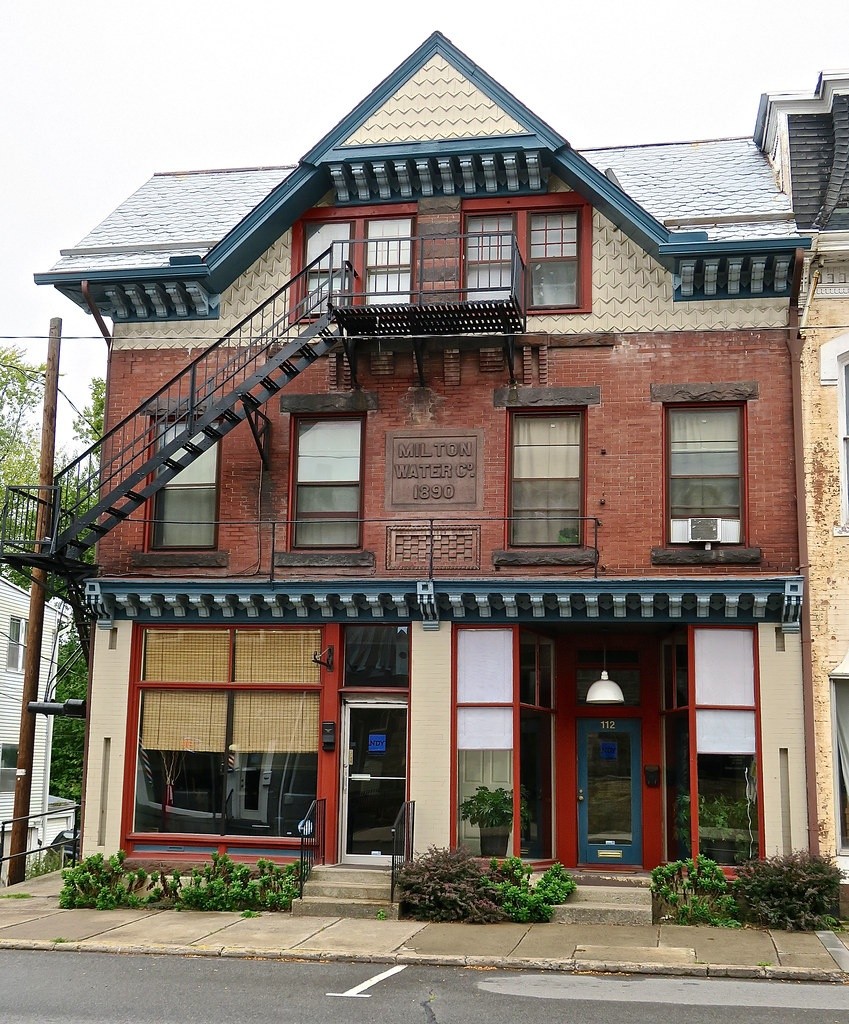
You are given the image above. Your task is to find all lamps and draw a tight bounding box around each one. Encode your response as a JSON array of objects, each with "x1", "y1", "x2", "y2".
[{"x1": 586, "y1": 625, "x2": 624, "y2": 703}]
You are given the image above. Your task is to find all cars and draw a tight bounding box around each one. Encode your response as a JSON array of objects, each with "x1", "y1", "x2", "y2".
[{"x1": 46, "y1": 830, "x2": 81, "y2": 861}]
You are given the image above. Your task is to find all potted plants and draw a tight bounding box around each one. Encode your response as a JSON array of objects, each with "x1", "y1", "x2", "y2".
[
  {"x1": 672, "y1": 794, "x2": 755, "y2": 863},
  {"x1": 459, "y1": 783, "x2": 531, "y2": 857}
]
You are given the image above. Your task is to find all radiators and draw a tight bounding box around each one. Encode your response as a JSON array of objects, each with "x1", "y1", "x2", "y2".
[{"x1": 671, "y1": 518, "x2": 740, "y2": 543}]
